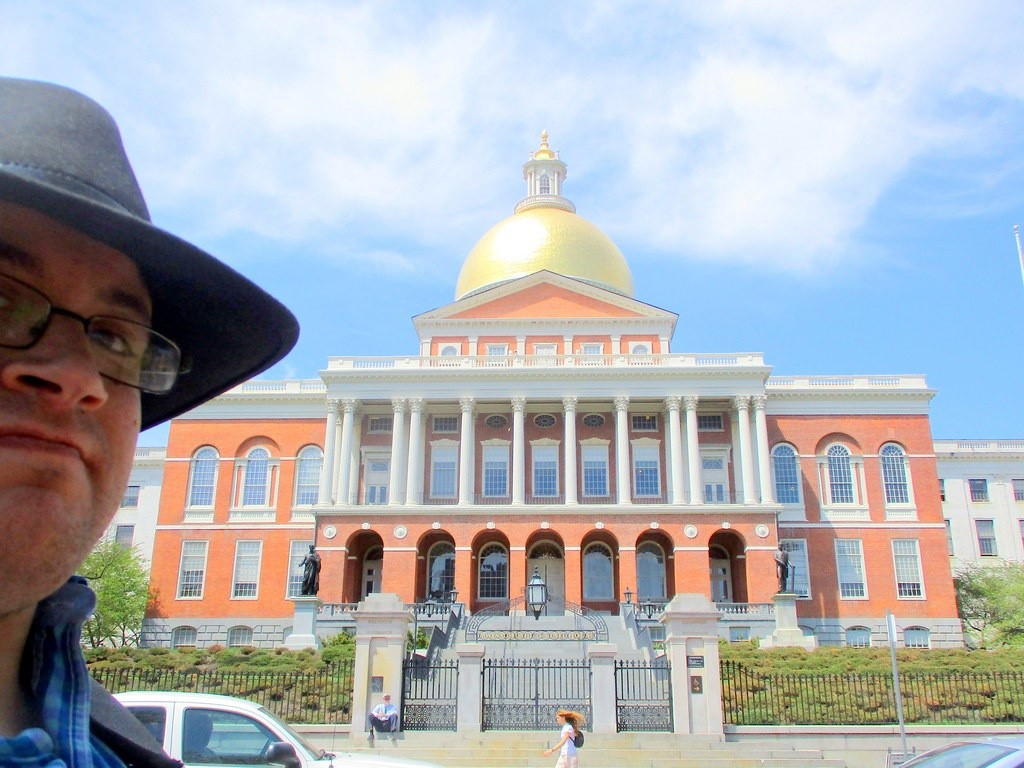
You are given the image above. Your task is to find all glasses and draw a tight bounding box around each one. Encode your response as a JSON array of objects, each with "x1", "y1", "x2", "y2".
[{"x1": 0, "y1": 272, "x2": 192, "y2": 396}]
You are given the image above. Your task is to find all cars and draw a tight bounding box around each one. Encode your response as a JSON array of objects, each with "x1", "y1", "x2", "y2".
[
  {"x1": 891, "y1": 742, "x2": 1024, "y2": 768},
  {"x1": 109, "y1": 690, "x2": 438, "y2": 768}
]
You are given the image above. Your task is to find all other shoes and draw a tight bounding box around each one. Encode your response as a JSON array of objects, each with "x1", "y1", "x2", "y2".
[
  {"x1": 367, "y1": 734, "x2": 374, "y2": 741},
  {"x1": 391, "y1": 737, "x2": 398, "y2": 741}
]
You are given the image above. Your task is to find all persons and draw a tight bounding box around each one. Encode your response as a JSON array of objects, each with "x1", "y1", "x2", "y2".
[
  {"x1": 541, "y1": 707, "x2": 587, "y2": 768},
  {"x1": 773, "y1": 541, "x2": 796, "y2": 594},
  {"x1": 1, "y1": 73, "x2": 298, "y2": 768},
  {"x1": 298, "y1": 544, "x2": 321, "y2": 596},
  {"x1": 366, "y1": 692, "x2": 399, "y2": 742}
]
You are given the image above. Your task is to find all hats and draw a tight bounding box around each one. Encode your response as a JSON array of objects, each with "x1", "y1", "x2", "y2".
[{"x1": 1, "y1": 75, "x2": 301, "y2": 437}]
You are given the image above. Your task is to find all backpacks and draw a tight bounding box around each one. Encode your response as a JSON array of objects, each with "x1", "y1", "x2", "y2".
[{"x1": 566, "y1": 724, "x2": 584, "y2": 748}]
